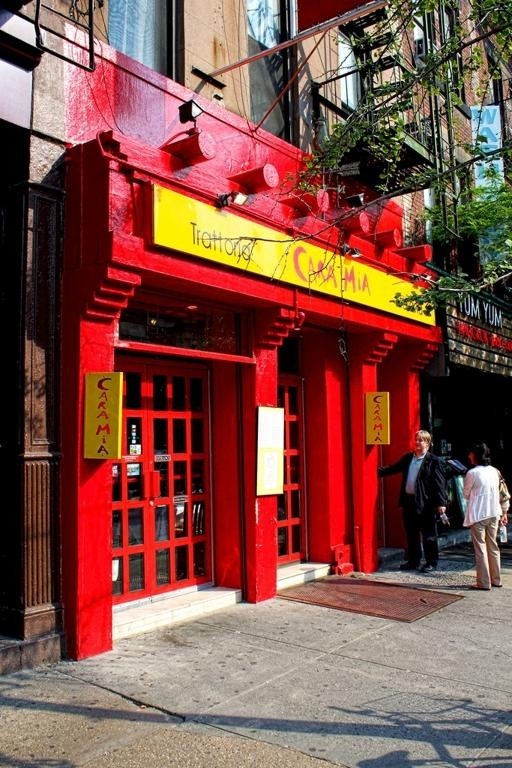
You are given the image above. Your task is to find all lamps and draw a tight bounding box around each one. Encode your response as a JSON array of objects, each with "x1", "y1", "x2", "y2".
[
  {"x1": 216, "y1": 191, "x2": 249, "y2": 208},
  {"x1": 343, "y1": 192, "x2": 364, "y2": 207},
  {"x1": 178, "y1": 99, "x2": 204, "y2": 127},
  {"x1": 340, "y1": 241, "x2": 363, "y2": 257}
]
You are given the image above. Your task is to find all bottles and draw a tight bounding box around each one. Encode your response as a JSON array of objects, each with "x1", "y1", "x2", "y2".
[
  {"x1": 499, "y1": 521, "x2": 509, "y2": 544},
  {"x1": 440, "y1": 510, "x2": 450, "y2": 528}
]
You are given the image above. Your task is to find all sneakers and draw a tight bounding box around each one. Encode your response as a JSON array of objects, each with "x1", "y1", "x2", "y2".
[{"x1": 469, "y1": 583, "x2": 503, "y2": 592}]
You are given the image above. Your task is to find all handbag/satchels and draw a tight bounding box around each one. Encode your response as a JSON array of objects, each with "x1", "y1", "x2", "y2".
[{"x1": 497, "y1": 469, "x2": 512, "y2": 504}]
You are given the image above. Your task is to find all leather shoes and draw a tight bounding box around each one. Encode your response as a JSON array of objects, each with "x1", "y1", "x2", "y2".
[{"x1": 398, "y1": 562, "x2": 436, "y2": 575}]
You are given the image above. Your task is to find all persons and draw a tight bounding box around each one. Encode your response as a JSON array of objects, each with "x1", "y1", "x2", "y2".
[
  {"x1": 378, "y1": 430, "x2": 450, "y2": 571},
  {"x1": 462, "y1": 442, "x2": 511, "y2": 590}
]
